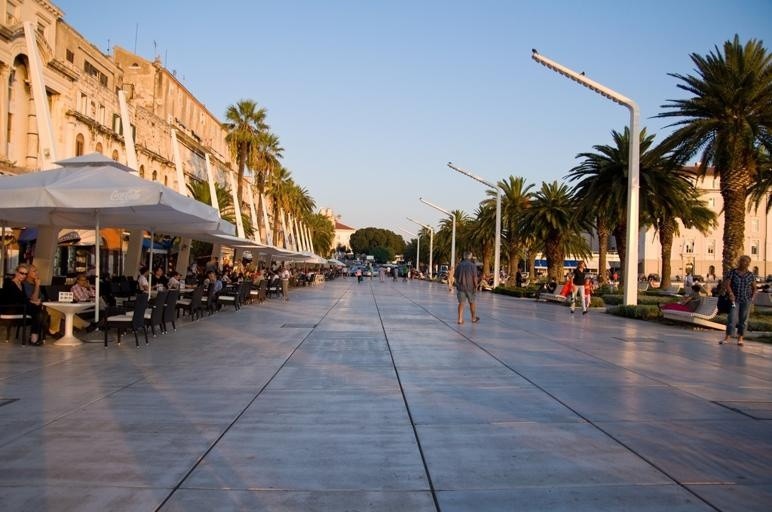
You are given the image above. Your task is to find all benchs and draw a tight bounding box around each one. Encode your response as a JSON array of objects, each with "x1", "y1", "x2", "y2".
[
  {"x1": 539, "y1": 285, "x2": 567, "y2": 302},
  {"x1": 662, "y1": 296, "x2": 727, "y2": 330},
  {"x1": 754, "y1": 293, "x2": 772, "y2": 307}
]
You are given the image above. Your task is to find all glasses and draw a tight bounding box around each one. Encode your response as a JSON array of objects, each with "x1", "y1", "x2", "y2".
[{"x1": 17, "y1": 271, "x2": 28, "y2": 275}]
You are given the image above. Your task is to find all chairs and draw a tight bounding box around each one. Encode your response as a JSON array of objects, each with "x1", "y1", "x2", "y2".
[{"x1": 0, "y1": 270, "x2": 313, "y2": 352}]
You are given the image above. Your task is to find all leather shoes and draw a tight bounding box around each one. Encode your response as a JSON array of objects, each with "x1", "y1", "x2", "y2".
[{"x1": 30, "y1": 320, "x2": 105, "y2": 346}]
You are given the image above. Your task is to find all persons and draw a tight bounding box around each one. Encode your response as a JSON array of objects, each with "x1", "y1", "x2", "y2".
[
  {"x1": 445, "y1": 264, "x2": 455, "y2": 292},
  {"x1": 516, "y1": 253, "x2": 772, "y2": 347},
  {"x1": 338, "y1": 265, "x2": 374, "y2": 282},
  {"x1": 455, "y1": 250, "x2": 480, "y2": 325},
  {"x1": 246, "y1": 264, "x2": 333, "y2": 300},
  {"x1": 71, "y1": 265, "x2": 106, "y2": 331},
  {"x1": 189, "y1": 261, "x2": 244, "y2": 309},
  {"x1": 137, "y1": 266, "x2": 192, "y2": 292},
  {"x1": 379, "y1": 266, "x2": 416, "y2": 282},
  {"x1": 3, "y1": 265, "x2": 44, "y2": 346}
]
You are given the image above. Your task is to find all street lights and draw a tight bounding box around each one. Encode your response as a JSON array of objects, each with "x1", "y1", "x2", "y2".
[
  {"x1": 396, "y1": 225, "x2": 420, "y2": 272},
  {"x1": 419, "y1": 196, "x2": 456, "y2": 275},
  {"x1": 529, "y1": 47, "x2": 644, "y2": 308},
  {"x1": 405, "y1": 216, "x2": 434, "y2": 279},
  {"x1": 445, "y1": 160, "x2": 502, "y2": 289}
]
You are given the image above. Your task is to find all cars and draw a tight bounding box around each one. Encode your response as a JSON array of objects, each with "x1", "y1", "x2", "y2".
[
  {"x1": 343, "y1": 260, "x2": 449, "y2": 279},
  {"x1": 585, "y1": 271, "x2": 597, "y2": 278}
]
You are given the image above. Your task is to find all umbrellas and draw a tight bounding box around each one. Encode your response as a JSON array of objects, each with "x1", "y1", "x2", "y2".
[{"x1": 0, "y1": 151, "x2": 222, "y2": 323}]
